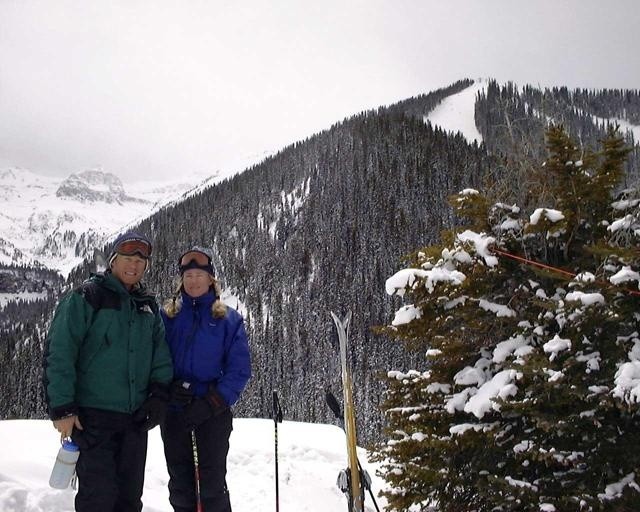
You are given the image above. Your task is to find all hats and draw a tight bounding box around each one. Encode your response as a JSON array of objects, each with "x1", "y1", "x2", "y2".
[
  {"x1": 110, "y1": 233, "x2": 151, "y2": 272},
  {"x1": 180, "y1": 259, "x2": 215, "y2": 277}
]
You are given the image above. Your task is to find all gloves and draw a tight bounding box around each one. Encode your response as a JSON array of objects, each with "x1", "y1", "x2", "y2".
[
  {"x1": 180, "y1": 380, "x2": 230, "y2": 428},
  {"x1": 170, "y1": 379, "x2": 194, "y2": 406},
  {"x1": 130, "y1": 384, "x2": 174, "y2": 433}
]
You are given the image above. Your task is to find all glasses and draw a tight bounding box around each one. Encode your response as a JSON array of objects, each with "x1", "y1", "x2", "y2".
[
  {"x1": 116, "y1": 238, "x2": 151, "y2": 258},
  {"x1": 180, "y1": 251, "x2": 210, "y2": 267}
]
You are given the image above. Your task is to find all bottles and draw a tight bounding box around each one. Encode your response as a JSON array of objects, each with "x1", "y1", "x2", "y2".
[{"x1": 49, "y1": 442, "x2": 81, "y2": 490}]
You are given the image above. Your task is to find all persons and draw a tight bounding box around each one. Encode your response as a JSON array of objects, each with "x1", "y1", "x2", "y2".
[
  {"x1": 150, "y1": 245, "x2": 253, "y2": 512},
  {"x1": 41, "y1": 230, "x2": 176, "y2": 511}
]
